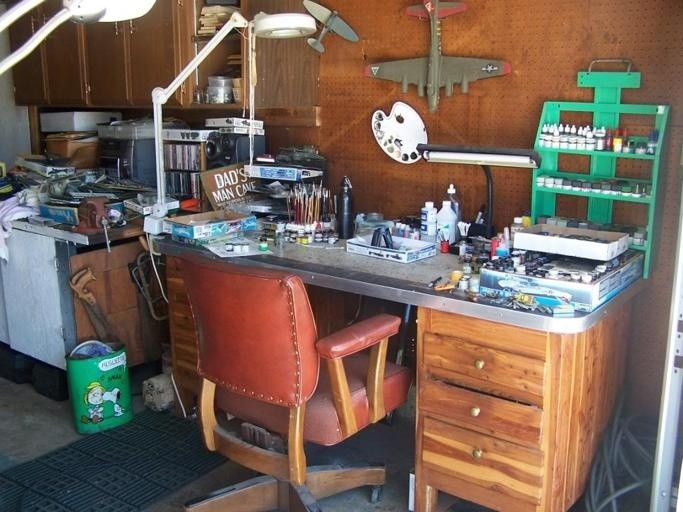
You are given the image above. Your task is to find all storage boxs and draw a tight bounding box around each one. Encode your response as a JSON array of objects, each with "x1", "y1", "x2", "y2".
[{"x1": 45, "y1": 132, "x2": 98, "y2": 169}]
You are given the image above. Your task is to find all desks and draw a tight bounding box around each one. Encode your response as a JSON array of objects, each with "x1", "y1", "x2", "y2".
[
  {"x1": 153, "y1": 232, "x2": 653, "y2": 512},
  {"x1": 1, "y1": 223, "x2": 167, "y2": 375}
]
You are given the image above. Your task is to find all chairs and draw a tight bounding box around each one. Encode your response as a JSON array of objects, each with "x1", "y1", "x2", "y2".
[{"x1": 181, "y1": 254, "x2": 411, "y2": 511}]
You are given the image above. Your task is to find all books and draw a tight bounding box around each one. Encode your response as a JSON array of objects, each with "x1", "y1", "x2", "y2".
[{"x1": 162, "y1": 143, "x2": 201, "y2": 201}]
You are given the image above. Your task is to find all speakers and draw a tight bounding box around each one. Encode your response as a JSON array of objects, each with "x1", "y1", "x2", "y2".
[{"x1": 204, "y1": 131, "x2": 266, "y2": 171}]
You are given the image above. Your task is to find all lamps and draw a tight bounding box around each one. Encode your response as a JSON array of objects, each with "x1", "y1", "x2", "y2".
[
  {"x1": 416, "y1": 143, "x2": 542, "y2": 239},
  {"x1": 142, "y1": 10, "x2": 319, "y2": 235}
]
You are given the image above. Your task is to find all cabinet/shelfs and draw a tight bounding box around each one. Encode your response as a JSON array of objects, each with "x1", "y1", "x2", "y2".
[
  {"x1": 165, "y1": 255, "x2": 365, "y2": 417},
  {"x1": 13, "y1": 0, "x2": 185, "y2": 108},
  {"x1": 414, "y1": 306, "x2": 633, "y2": 512},
  {"x1": 190, "y1": 0, "x2": 250, "y2": 110}
]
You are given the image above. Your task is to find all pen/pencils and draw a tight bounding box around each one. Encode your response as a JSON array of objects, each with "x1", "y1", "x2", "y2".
[
  {"x1": 428, "y1": 277, "x2": 442, "y2": 287},
  {"x1": 288, "y1": 180, "x2": 332, "y2": 223}
]
